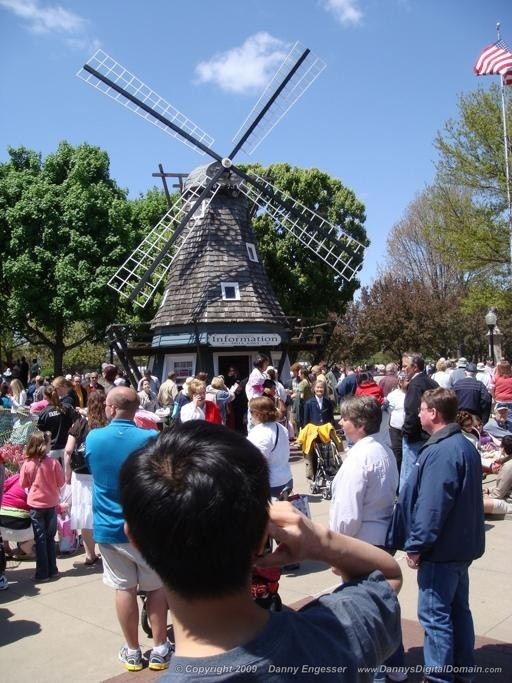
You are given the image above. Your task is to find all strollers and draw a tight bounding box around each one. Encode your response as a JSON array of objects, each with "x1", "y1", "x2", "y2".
[{"x1": 309, "y1": 422, "x2": 343, "y2": 501}]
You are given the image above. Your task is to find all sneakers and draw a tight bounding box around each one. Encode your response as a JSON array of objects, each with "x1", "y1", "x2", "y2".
[
  {"x1": 148, "y1": 649, "x2": 173, "y2": 671},
  {"x1": 116, "y1": 644, "x2": 142, "y2": 671},
  {"x1": 0, "y1": 577, "x2": 8, "y2": 589},
  {"x1": 31, "y1": 576, "x2": 49, "y2": 581}
]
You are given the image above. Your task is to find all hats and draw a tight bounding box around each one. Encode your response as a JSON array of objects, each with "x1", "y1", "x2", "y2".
[
  {"x1": 457, "y1": 362, "x2": 483, "y2": 374},
  {"x1": 477, "y1": 362, "x2": 486, "y2": 371},
  {"x1": 457, "y1": 357, "x2": 466, "y2": 367},
  {"x1": 495, "y1": 403, "x2": 508, "y2": 411},
  {"x1": 102, "y1": 362, "x2": 119, "y2": 377}
]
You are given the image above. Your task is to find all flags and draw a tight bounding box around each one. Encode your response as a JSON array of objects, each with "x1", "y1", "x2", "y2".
[{"x1": 473, "y1": 39, "x2": 512, "y2": 85}]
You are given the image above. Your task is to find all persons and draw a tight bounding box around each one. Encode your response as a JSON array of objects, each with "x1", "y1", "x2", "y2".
[
  {"x1": 0, "y1": 355, "x2": 105, "y2": 592},
  {"x1": 133, "y1": 394, "x2": 166, "y2": 432},
  {"x1": 97, "y1": 362, "x2": 130, "y2": 396},
  {"x1": 329, "y1": 361, "x2": 402, "y2": 395},
  {"x1": 402, "y1": 351, "x2": 512, "y2": 683},
  {"x1": 85, "y1": 387, "x2": 176, "y2": 671},
  {"x1": 290, "y1": 360, "x2": 334, "y2": 429},
  {"x1": 137, "y1": 379, "x2": 160, "y2": 414},
  {"x1": 329, "y1": 394, "x2": 408, "y2": 683},
  {"x1": 157, "y1": 371, "x2": 179, "y2": 431},
  {"x1": 175, "y1": 368, "x2": 247, "y2": 424},
  {"x1": 137, "y1": 369, "x2": 160, "y2": 396},
  {"x1": 118, "y1": 420, "x2": 402, "y2": 682},
  {"x1": 247, "y1": 397, "x2": 301, "y2": 572},
  {"x1": 245, "y1": 352, "x2": 290, "y2": 403}
]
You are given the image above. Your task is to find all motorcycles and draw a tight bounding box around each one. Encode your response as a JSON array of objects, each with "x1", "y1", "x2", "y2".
[
  {"x1": 142, "y1": 537, "x2": 283, "y2": 635},
  {"x1": 271, "y1": 486, "x2": 311, "y2": 570}
]
